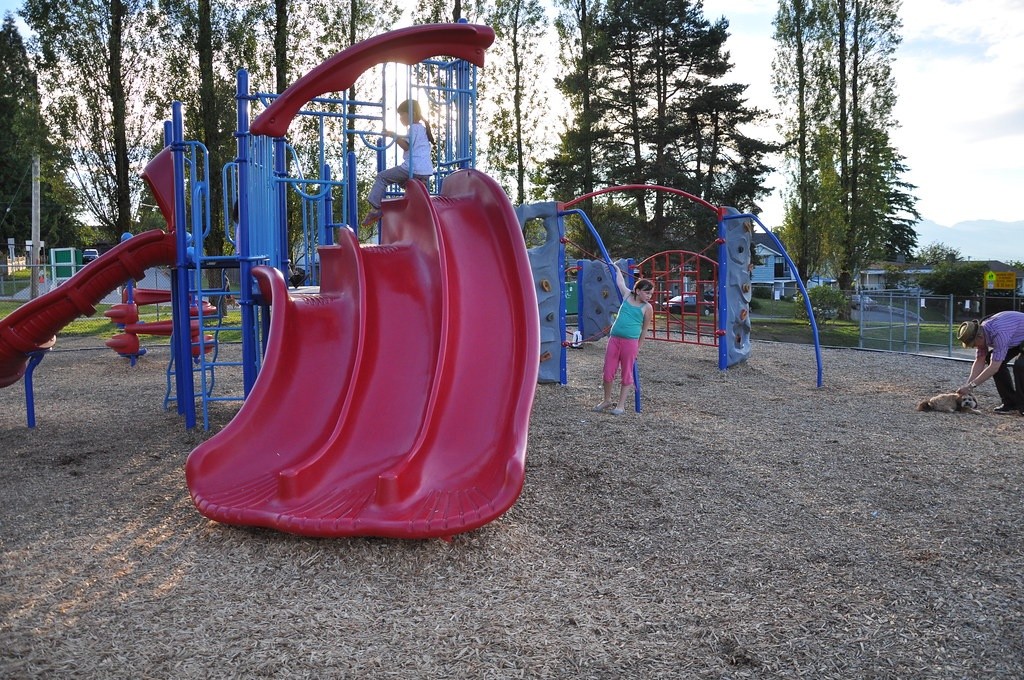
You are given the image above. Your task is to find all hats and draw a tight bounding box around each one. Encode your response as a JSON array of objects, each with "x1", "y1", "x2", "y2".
[{"x1": 956, "y1": 318, "x2": 979, "y2": 349}]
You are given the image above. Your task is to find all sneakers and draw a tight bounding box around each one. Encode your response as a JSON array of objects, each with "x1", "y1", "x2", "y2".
[
  {"x1": 361, "y1": 208, "x2": 384, "y2": 227},
  {"x1": 1020, "y1": 409, "x2": 1024, "y2": 415},
  {"x1": 993, "y1": 404, "x2": 1019, "y2": 414}
]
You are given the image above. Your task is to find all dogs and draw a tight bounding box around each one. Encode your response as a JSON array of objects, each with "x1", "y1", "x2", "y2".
[
  {"x1": 916, "y1": 392, "x2": 982, "y2": 415},
  {"x1": 226, "y1": 299, "x2": 239, "y2": 309}
]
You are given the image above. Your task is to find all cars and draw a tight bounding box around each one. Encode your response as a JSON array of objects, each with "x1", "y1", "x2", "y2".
[
  {"x1": 663, "y1": 295, "x2": 714, "y2": 316},
  {"x1": 82, "y1": 249, "x2": 99, "y2": 264},
  {"x1": 846, "y1": 295, "x2": 878, "y2": 311}
]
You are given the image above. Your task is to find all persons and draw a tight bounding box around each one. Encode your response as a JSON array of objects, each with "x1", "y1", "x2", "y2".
[
  {"x1": 362, "y1": 98, "x2": 436, "y2": 226},
  {"x1": 955, "y1": 311, "x2": 1024, "y2": 417},
  {"x1": 591, "y1": 260, "x2": 654, "y2": 415}
]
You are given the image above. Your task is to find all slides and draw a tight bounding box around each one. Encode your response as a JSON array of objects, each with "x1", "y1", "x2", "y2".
[
  {"x1": 186, "y1": 167, "x2": 540, "y2": 533},
  {"x1": 0, "y1": 223, "x2": 173, "y2": 388}
]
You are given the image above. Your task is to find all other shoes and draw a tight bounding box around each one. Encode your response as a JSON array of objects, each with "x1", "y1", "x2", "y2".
[
  {"x1": 591, "y1": 400, "x2": 611, "y2": 411},
  {"x1": 611, "y1": 407, "x2": 626, "y2": 414}
]
